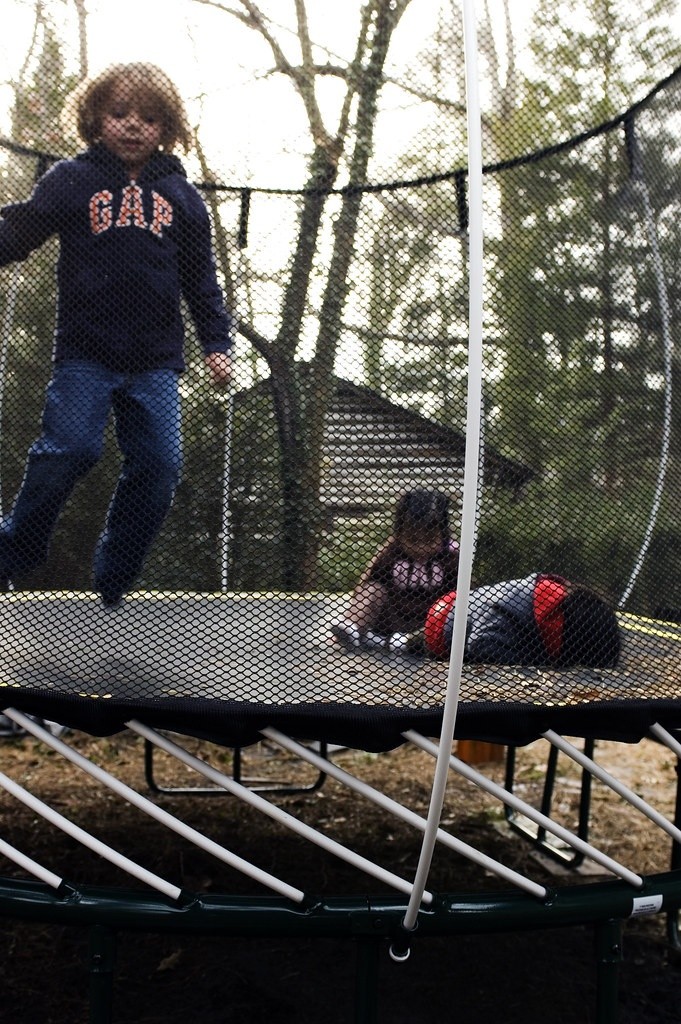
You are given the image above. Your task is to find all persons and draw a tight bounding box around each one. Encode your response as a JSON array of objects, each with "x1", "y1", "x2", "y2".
[
  {"x1": 332, "y1": 490, "x2": 621, "y2": 669},
  {"x1": 0, "y1": 61, "x2": 235, "y2": 609}
]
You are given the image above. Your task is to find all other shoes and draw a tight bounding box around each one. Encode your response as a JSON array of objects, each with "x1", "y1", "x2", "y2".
[
  {"x1": 331, "y1": 621, "x2": 361, "y2": 651},
  {"x1": 384, "y1": 633, "x2": 407, "y2": 653}
]
